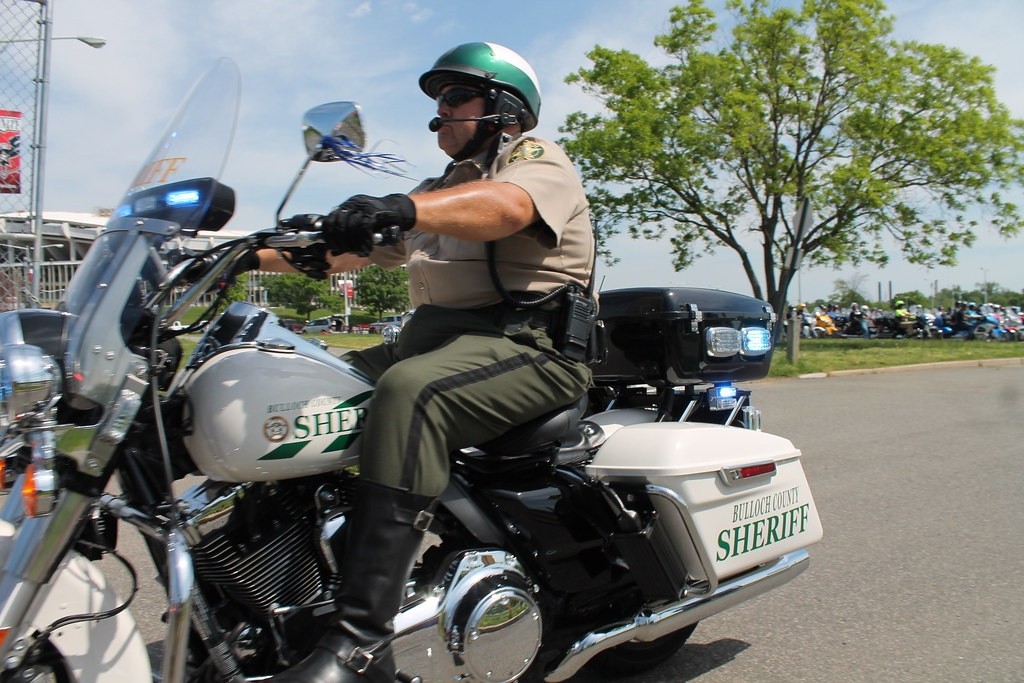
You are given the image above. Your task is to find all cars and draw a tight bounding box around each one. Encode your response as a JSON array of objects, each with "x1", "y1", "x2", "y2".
[
  {"x1": 302, "y1": 313, "x2": 352, "y2": 334},
  {"x1": 369, "y1": 314, "x2": 402, "y2": 334},
  {"x1": 279, "y1": 318, "x2": 304, "y2": 334}
]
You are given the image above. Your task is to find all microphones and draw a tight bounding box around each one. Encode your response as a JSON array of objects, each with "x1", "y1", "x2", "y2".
[{"x1": 429, "y1": 113, "x2": 509, "y2": 133}]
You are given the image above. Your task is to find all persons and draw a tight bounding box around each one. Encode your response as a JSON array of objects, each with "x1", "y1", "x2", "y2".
[
  {"x1": 169, "y1": 41, "x2": 601, "y2": 683},
  {"x1": 783, "y1": 300, "x2": 1024, "y2": 345}
]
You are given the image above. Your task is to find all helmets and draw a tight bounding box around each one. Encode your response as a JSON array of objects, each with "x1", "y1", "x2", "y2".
[
  {"x1": 795, "y1": 298, "x2": 1023, "y2": 319},
  {"x1": 418, "y1": 42, "x2": 542, "y2": 133}
]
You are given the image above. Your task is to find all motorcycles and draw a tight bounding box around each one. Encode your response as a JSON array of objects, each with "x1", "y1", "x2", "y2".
[
  {"x1": 783, "y1": 303, "x2": 1024, "y2": 344},
  {"x1": 0, "y1": 51, "x2": 825, "y2": 683}
]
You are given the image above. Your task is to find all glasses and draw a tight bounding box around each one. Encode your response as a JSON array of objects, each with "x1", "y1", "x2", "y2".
[{"x1": 436, "y1": 87, "x2": 490, "y2": 107}]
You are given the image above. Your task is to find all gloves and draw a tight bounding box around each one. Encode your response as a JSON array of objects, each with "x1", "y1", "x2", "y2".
[
  {"x1": 322, "y1": 194, "x2": 417, "y2": 256},
  {"x1": 206, "y1": 248, "x2": 260, "y2": 284}
]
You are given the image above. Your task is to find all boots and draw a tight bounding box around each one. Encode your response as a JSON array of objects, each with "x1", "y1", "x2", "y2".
[{"x1": 267, "y1": 476, "x2": 439, "y2": 683}]
